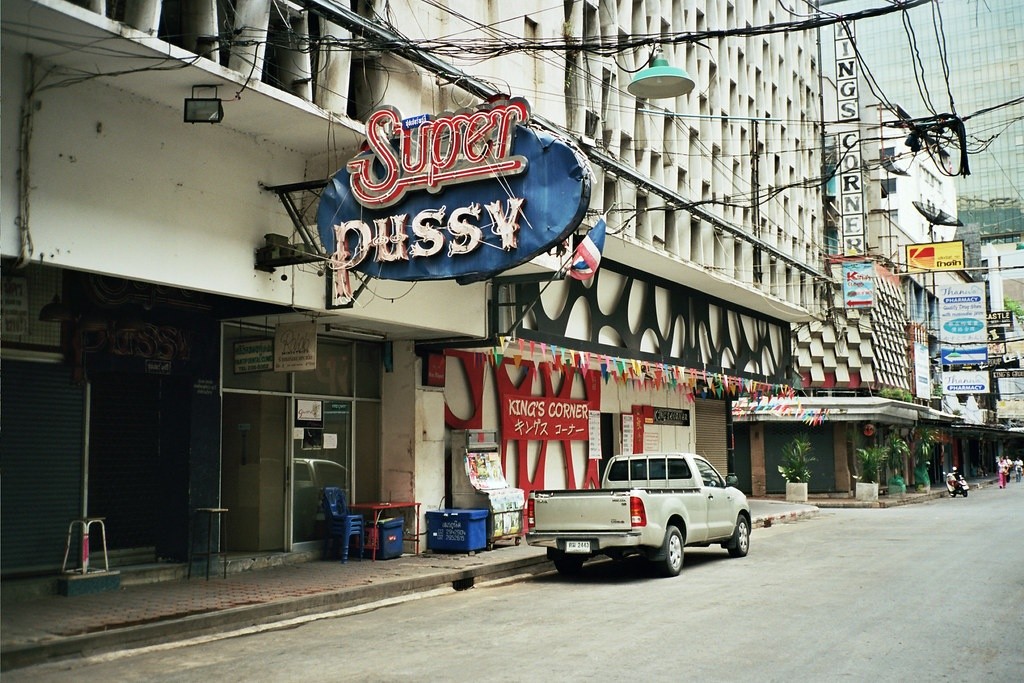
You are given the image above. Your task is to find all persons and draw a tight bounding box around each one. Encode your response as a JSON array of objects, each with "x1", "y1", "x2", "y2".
[
  {"x1": 998, "y1": 456, "x2": 1009, "y2": 488},
  {"x1": 1014, "y1": 457, "x2": 1023, "y2": 483},
  {"x1": 1003, "y1": 456, "x2": 1013, "y2": 483}
]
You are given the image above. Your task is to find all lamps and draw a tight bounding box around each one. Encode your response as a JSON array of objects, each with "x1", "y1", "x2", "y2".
[
  {"x1": 627, "y1": 43, "x2": 695, "y2": 98},
  {"x1": 183, "y1": 84, "x2": 225, "y2": 125}
]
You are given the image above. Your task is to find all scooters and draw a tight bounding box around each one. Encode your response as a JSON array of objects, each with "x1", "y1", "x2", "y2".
[{"x1": 942, "y1": 467, "x2": 969, "y2": 497}]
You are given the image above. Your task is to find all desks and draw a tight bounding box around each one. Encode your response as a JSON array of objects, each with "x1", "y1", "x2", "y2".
[{"x1": 347, "y1": 503, "x2": 420, "y2": 562}]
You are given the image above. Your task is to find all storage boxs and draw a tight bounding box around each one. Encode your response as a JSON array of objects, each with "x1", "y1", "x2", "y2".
[
  {"x1": 427, "y1": 508, "x2": 488, "y2": 551},
  {"x1": 353, "y1": 518, "x2": 405, "y2": 560}
]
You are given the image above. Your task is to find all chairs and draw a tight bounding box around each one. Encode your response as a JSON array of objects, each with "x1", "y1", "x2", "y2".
[{"x1": 321, "y1": 485, "x2": 365, "y2": 564}]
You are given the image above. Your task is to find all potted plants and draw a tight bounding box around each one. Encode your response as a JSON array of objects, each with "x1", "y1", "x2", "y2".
[
  {"x1": 912, "y1": 426, "x2": 940, "y2": 493},
  {"x1": 884, "y1": 432, "x2": 913, "y2": 498},
  {"x1": 776, "y1": 431, "x2": 817, "y2": 501},
  {"x1": 843, "y1": 428, "x2": 891, "y2": 501}
]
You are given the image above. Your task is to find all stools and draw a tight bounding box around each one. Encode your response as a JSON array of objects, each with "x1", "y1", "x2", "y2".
[
  {"x1": 187, "y1": 507, "x2": 230, "y2": 580},
  {"x1": 61, "y1": 516, "x2": 109, "y2": 575}
]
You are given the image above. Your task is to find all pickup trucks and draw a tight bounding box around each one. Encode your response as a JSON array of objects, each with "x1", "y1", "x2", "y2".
[
  {"x1": 525, "y1": 451, "x2": 754, "y2": 578},
  {"x1": 188, "y1": 457, "x2": 378, "y2": 533}
]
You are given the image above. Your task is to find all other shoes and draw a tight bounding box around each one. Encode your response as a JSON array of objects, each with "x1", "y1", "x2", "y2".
[{"x1": 1000, "y1": 485, "x2": 1002, "y2": 488}]
